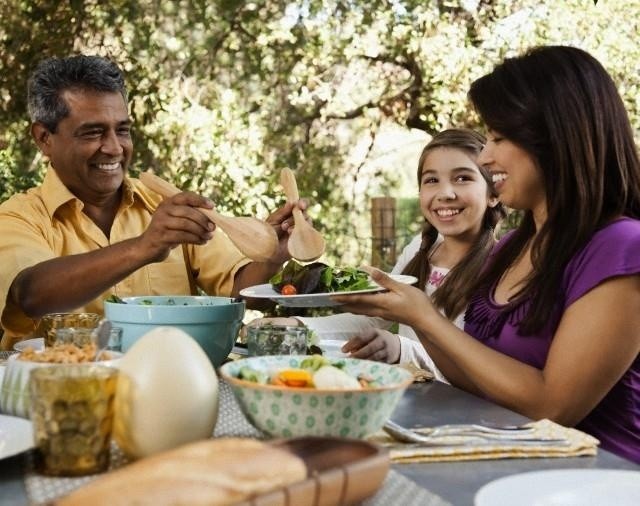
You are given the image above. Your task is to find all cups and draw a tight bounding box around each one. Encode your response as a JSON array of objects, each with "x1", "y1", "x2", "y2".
[
  {"x1": 247, "y1": 322, "x2": 309, "y2": 356},
  {"x1": 27, "y1": 363, "x2": 122, "y2": 475},
  {"x1": 40, "y1": 311, "x2": 100, "y2": 348}
]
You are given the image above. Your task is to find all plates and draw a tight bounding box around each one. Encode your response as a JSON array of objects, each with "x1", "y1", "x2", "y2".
[
  {"x1": 0, "y1": 409, "x2": 35, "y2": 463},
  {"x1": 11, "y1": 333, "x2": 45, "y2": 354},
  {"x1": 238, "y1": 272, "x2": 420, "y2": 309}
]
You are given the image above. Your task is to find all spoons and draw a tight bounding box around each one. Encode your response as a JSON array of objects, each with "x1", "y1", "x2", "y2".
[{"x1": 383, "y1": 419, "x2": 573, "y2": 448}]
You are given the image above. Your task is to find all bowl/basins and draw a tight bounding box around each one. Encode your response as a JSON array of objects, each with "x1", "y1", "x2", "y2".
[
  {"x1": 0, "y1": 350, "x2": 126, "y2": 420},
  {"x1": 103, "y1": 291, "x2": 247, "y2": 371},
  {"x1": 217, "y1": 351, "x2": 416, "y2": 440}
]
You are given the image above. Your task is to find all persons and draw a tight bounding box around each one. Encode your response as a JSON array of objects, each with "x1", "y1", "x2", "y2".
[
  {"x1": 236, "y1": 128, "x2": 507, "y2": 387},
  {"x1": 329, "y1": 46, "x2": 640, "y2": 464},
  {"x1": 0, "y1": 53, "x2": 310, "y2": 358}
]
